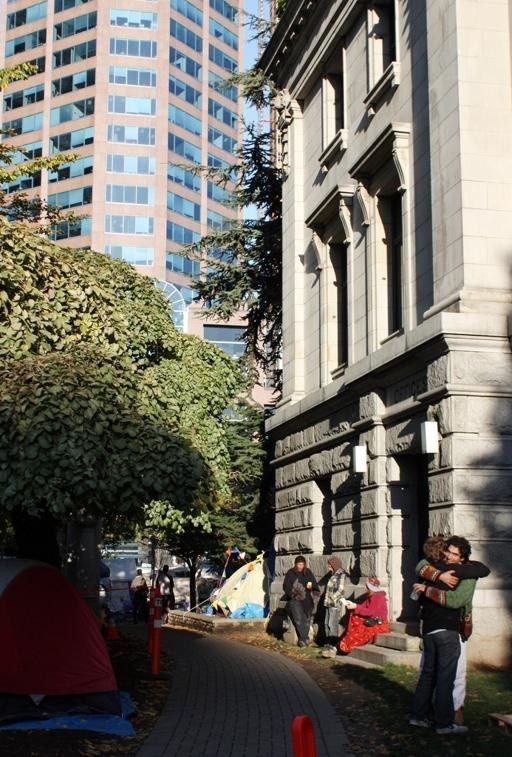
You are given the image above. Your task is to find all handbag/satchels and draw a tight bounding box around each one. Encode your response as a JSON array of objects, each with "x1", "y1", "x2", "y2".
[{"x1": 292, "y1": 573, "x2": 306, "y2": 600}]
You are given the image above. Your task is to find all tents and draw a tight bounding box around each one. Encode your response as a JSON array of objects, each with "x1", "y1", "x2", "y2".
[
  {"x1": 203, "y1": 548, "x2": 272, "y2": 618},
  {"x1": 2, "y1": 555, "x2": 135, "y2": 738}
]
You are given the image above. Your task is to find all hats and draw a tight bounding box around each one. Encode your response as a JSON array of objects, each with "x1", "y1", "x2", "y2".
[{"x1": 366, "y1": 577, "x2": 382, "y2": 592}]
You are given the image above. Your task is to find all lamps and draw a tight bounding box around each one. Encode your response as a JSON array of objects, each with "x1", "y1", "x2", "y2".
[
  {"x1": 420, "y1": 420, "x2": 442, "y2": 454},
  {"x1": 353, "y1": 445, "x2": 370, "y2": 473}
]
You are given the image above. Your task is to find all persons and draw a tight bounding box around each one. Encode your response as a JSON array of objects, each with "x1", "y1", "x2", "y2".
[
  {"x1": 196, "y1": 577, "x2": 211, "y2": 607},
  {"x1": 100, "y1": 614, "x2": 121, "y2": 639},
  {"x1": 321, "y1": 575, "x2": 390, "y2": 658},
  {"x1": 316, "y1": 556, "x2": 346, "y2": 654},
  {"x1": 406, "y1": 534, "x2": 491, "y2": 734},
  {"x1": 130, "y1": 562, "x2": 177, "y2": 626},
  {"x1": 410, "y1": 533, "x2": 477, "y2": 726},
  {"x1": 281, "y1": 554, "x2": 321, "y2": 647}
]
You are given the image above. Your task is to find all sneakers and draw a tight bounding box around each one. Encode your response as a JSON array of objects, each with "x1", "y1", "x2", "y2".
[
  {"x1": 318, "y1": 642, "x2": 338, "y2": 659},
  {"x1": 453, "y1": 707, "x2": 465, "y2": 726},
  {"x1": 436, "y1": 722, "x2": 469, "y2": 735},
  {"x1": 409, "y1": 716, "x2": 434, "y2": 728},
  {"x1": 298, "y1": 637, "x2": 310, "y2": 648}
]
